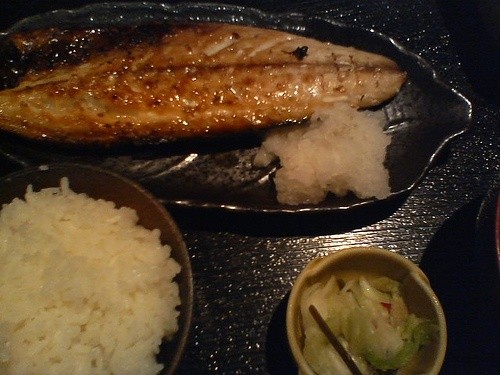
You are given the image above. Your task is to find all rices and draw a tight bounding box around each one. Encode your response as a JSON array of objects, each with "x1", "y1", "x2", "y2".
[
  {"x1": 253, "y1": 103, "x2": 393, "y2": 206},
  {"x1": 0, "y1": 177, "x2": 182, "y2": 375}
]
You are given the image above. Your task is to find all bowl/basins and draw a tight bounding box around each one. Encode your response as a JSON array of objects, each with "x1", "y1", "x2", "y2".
[
  {"x1": 1, "y1": 162, "x2": 194, "y2": 375},
  {"x1": 286, "y1": 248, "x2": 447, "y2": 375}
]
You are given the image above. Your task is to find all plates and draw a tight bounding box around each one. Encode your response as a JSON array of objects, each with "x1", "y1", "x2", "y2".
[
  {"x1": 478, "y1": 170, "x2": 500, "y2": 301},
  {"x1": 1, "y1": 1, "x2": 473, "y2": 216}
]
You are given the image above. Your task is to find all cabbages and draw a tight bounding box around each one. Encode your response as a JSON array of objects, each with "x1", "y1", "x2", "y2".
[{"x1": 299, "y1": 272, "x2": 438, "y2": 375}]
